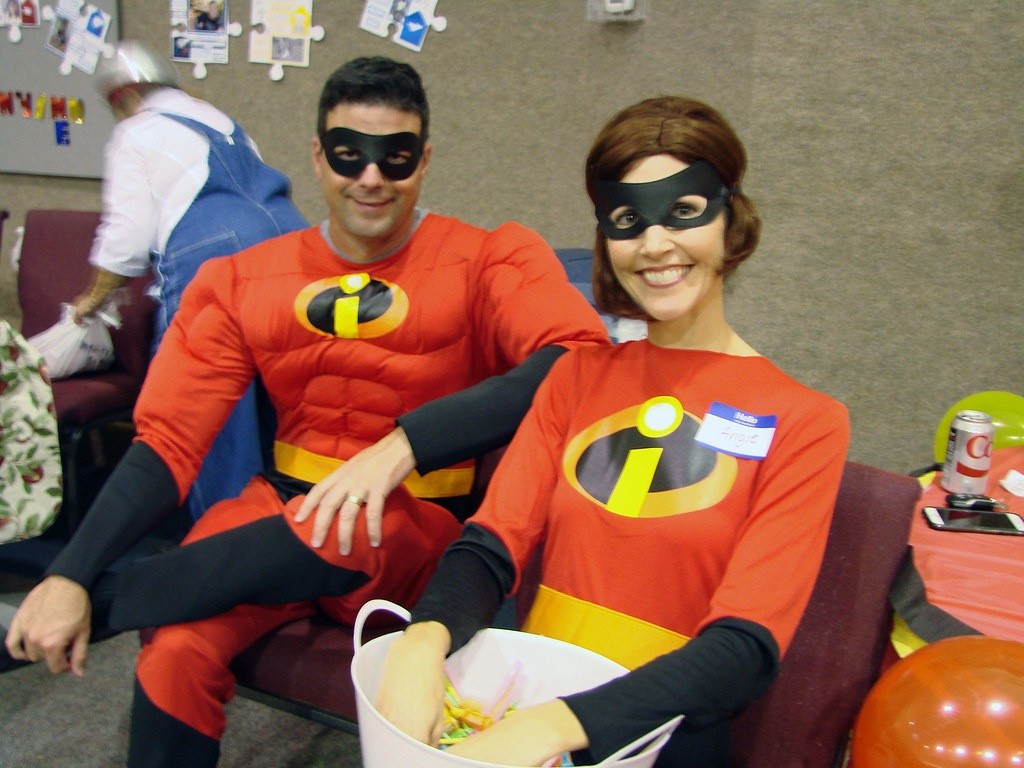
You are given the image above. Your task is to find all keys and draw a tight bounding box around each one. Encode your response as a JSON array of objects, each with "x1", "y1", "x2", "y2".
[{"x1": 946, "y1": 493, "x2": 998, "y2": 511}]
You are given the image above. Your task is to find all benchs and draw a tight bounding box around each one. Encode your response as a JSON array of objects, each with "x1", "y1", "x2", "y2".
[{"x1": 139, "y1": 445, "x2": 923, "y2": 767}]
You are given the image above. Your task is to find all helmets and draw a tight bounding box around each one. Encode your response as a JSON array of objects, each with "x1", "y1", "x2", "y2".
[{"x1": 94, "y1": 37, "x2": 180, "y2": 98}]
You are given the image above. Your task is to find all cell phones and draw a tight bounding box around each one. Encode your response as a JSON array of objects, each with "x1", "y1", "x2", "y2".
[{"x1": 923, "y1": 506, "x2": 1024, "y2": 536}]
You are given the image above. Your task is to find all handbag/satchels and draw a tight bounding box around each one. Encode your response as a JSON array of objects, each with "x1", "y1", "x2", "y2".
[{"x1": 27, "y1": 300, "x2": 121, "y2": 378}]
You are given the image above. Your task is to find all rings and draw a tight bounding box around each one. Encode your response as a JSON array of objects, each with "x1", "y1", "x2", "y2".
[{"x1": 346, "y1": 494, "x2": 363, "y2": 505}]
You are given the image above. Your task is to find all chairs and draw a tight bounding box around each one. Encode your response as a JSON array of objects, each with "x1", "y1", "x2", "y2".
[{"x1": 16, "y1": 210, "x2": 162, "y2": 542}]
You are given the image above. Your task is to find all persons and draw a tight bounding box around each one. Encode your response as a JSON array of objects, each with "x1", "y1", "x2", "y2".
[
  {"x1": 5, "y1": 1, "x2": 19, "y2": 18},
  {"x1": 74, "y1": 43, "x2": 313, "y2": 525},
  {"x1": 195, "y1": 1, "x2": 223, "y2": 32},
  {"x1": 375, "y1": 97, "x2": 850, "y2": 768},
  {"x1": 0, "y1": 54, "x2": 613, "y2": 767}
]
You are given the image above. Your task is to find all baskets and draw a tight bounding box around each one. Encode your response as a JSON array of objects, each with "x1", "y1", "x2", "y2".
[{"x1": 351, "y1": 598, "x2": 684, "y2": 767}]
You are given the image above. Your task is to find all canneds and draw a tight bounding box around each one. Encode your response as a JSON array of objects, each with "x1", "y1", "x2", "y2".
[{"x1": 940, "y1": 409, "x2": 994, "y2": 495}]
